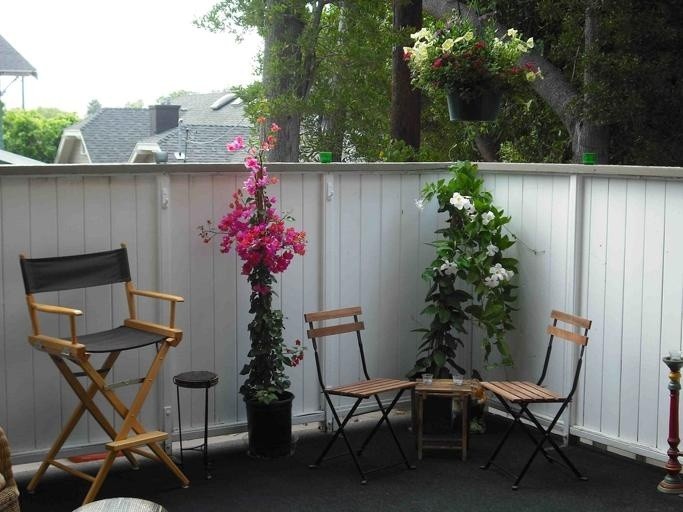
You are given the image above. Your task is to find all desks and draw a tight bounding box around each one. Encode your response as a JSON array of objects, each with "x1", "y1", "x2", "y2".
[{"x1": 412, "y1": 374, "x2": 475, "y2": 464}]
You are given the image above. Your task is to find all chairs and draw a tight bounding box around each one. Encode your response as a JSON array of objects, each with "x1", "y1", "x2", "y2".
[
  {"x1": 12, "y1": 239, "x2": 192, "y2": 508},
  {"x1": 302, "y1": 303, "x2": 415, "y2": 485},
  {"x1": 478, "y1": 308, "x2": 594, "y2": 491}
]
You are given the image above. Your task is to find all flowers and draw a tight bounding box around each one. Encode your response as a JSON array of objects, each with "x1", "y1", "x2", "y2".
[
  {"x1": 197, "y1": 114, "x2": 312, "y2": 404},
  {"x1": 399, "y1": 154, "x2": 525, "y2": 383}
]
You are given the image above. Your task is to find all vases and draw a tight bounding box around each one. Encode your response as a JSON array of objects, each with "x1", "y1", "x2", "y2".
[
  {"x1": 238, "y1": 391, "x2": 294, "y2": 459},
  {"x1": 406, "y1": 366, "x2": 456, "y2": 458}
]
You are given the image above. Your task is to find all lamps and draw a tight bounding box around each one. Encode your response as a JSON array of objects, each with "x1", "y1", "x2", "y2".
[{"x1": 655, "y1": 349, "x2": 682, "y2": 496}]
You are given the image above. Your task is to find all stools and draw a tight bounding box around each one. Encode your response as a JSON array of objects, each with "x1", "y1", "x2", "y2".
[{"x1": 170, "y1": 370, "x2": 220, "y2": 489}]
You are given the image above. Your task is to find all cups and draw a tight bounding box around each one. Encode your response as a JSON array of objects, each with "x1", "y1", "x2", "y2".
[
  {"x1": 151, "y1": 151, "x2": 166, "y2": 164},
  {"x1": 452, "y1": 375, "x2": 463, "y2": 386},
  {"x1": 422, "y1": 372, "x2": 432, "y2": 385},
  {"x1": 318, "y1": 150, "x2": 332, "y2": 162},
  {"x1": 581, "y1": 152, "x2": 594, "y2": 165}
]
